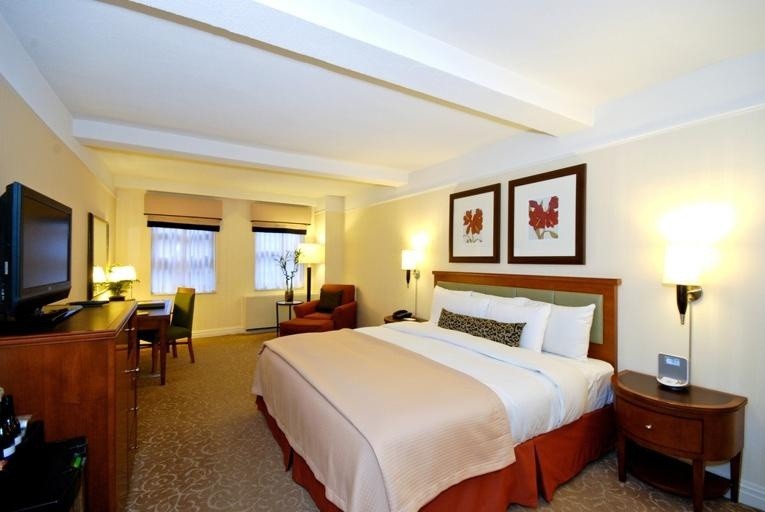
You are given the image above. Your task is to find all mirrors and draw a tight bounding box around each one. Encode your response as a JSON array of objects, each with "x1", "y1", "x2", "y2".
[{"x1": 86, "y1": 211, "x2": 110, "y2": 298}]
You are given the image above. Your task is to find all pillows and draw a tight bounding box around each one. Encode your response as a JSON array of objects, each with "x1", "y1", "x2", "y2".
[
  {"x1": 529, "y1": 298, "x2": 596, "y2": 363},
  {"x1": 437, "y1": 288, "x2": 490, "y2": 323},
  {"x1": 429, "y1": 284, "x2": 473, "y2": 323},
  {"x1": 471, "y1": 289, "x2": 528, "y2": 305},
  {"x1": 316, "y1": 286, "x2": 344, "y2": 314},
  {"x1": 489, "y1": 295, "x2": 553, "y2": 356},
  {"x1": 437, "y1": 306, "x2": 527, "y2": 349}
]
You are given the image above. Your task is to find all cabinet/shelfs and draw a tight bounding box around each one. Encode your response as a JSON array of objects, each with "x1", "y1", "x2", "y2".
[
  {"x1": 0, "y1": 416, "x2": 96, "y2": 512},
  {"x1": 1, "y1": 299, "x2": 139, "y2": 512}
]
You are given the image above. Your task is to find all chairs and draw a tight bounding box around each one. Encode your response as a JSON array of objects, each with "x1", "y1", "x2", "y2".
[
  {"x1": 278, "y1": 282, "x2": 358, "y2": 330},
  {"x1": 137, "y1": 285, "x2": 199, "y2": 376}
]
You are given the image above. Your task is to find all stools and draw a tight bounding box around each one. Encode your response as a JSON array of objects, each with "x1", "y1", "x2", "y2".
[{"x1": 279, "y1": 318, "x2": 334, "y2": 334}]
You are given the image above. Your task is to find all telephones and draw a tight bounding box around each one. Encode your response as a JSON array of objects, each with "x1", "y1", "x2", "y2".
[{"x1": 392, "y1": 310, "x2": 412, "y2": 320}]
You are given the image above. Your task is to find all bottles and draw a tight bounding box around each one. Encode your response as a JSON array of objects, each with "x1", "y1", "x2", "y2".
[
  {"x1": 1, "y1": 402, "x2": 19, "y2": 460},
  {"x1": 7, "y1": 395, "x2": 24, "y2": 448}
]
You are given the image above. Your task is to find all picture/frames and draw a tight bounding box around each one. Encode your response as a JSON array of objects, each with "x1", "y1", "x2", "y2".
[
  {"x1": 508, "y1": 162, "x2": 589, "y2": 266},
  {"x1": 448, "y1": 182, "x2": 501, "y2": 264}
]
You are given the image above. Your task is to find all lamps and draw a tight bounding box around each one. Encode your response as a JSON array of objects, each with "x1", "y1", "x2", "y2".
[
  {"x1": 92, "y1": 261, "x2": 106, "y2": 294},
  {"x1": 402, "y1": 247, "x2": 423, "y2": 317},
  {"x1": 111, "y1": 265, "x2": 138, "y2": 300},
  {"x1": 296, "y1": 240, "x2": 325, "y2": 302},
  {"x1": 658, "y1": 239, "x2": 706, "y2": 390}
]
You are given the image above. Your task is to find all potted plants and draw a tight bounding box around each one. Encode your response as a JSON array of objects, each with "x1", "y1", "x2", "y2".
[{"x1": 267, "y1": 247, "x2": 303, "y2": 302}]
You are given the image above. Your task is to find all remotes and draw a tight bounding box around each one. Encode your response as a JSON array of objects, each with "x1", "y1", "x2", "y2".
[{"x1": 69, "y1": 301, "x2": 109, "y2": 306}]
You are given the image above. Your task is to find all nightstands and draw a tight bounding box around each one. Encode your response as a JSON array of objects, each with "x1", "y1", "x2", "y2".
[
  {"x1": 607, "y1": 370, "x2": 750, "y2": 512},
  {"x1": 382, "y1": 312, "x2": 428, "y2": 325}
]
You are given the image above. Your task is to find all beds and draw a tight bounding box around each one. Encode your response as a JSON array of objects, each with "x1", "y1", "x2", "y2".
[{"x1": 248, "y1": 269, "x2": 624, "y2": 512}]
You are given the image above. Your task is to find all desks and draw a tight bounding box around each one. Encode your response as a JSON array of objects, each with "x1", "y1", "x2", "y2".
[
  {"x1": 275, "y1": 303, "x2": 301, "y2": 336},
  {"x1": 125, "y1": 296, "x2": 172, "y2": 388}
]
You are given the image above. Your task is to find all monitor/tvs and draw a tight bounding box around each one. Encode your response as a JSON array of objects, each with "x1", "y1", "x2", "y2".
[{"x1": 0, "y1": 181, "x2": 84, "y2": 337}]
